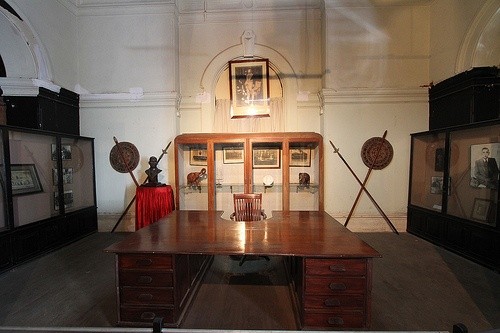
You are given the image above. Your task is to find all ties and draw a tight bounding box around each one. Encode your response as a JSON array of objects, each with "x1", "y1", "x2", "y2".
[{"x1": 484, "y1": 159, "x2": 488, "y2": 165}]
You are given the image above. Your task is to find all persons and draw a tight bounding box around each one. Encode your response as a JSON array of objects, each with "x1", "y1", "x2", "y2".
[
  {"x1": 245, "y1": 69, "x2": 255, "y2": 104},
  {"x1": 145, "y1": 157, "x2": 162, "y2": 183},
  {"x1": 474, "y1": 148, "x2": 499, "y2": 188}
]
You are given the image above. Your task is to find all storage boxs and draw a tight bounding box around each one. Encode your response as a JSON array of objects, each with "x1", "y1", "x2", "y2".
[
  {"x1": 428, "y1": 65, "x2": 500, "y2": 132},
  {"x1": 0, "y1": 77, "x2": 80, "y2": 135}
]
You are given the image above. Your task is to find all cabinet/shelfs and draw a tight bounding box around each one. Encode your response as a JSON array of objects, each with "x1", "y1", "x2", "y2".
[
  {"x1": 0, "y1": 124, "x2": 98, "y2": 274},
  {"x1": 174, "y1": 132, "x2": 324, "y2": 210},
  {"x1": 406, "y1": 119, "x2": 500, "y2": 273}
]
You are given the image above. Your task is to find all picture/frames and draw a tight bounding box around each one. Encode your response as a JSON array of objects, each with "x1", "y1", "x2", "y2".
[
  {"x1": 228, "y1": 58, "x2": 270, "y2": 119},
  {"x1": 469, "y1": 142, "x2": 500, "y2": 191},
  {"x1": 288, "y1": 146, "x2": 311, "y2": 167},
  {"x1": 54, "y1": 189, "x2": 74, "y2": 211},
  {"x1": 52, "y1": 167, "x2": 73, "y2": 185},
  {"x1": 0, "y1": 163, "x2": 43, "y2": 197},
  {"x1": 51, "y1": 143, "x2": 72, "y2": 161},
  {"x1": 434, "y1": 147, "x2": 451, "y2": 172},
  {"x1": 223, "y1": 146, "x2": 245, "y2": 164},
  {"x1": 189, "y1": 147, "x2": 215, "y2": 166},
  {"x1": 471, "y1": 198, "x2": 494, "y2": 222},
  {"x1": 252, "y1": 146, "x2": 281, "y2": 169},
  {"x1": 430, "y1": 176, "x2": 451, "y2": 194}
]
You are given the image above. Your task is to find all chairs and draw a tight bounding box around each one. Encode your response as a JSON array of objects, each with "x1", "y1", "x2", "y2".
[{"x1": 230, "y1": 192, "x2": 270, "y2": 266}]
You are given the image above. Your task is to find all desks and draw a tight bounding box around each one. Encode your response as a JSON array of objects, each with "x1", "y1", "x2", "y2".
[
  {"x1": 103, "y1": 210, "x2": 384, "y2": 331},
  {"x1": 135, "y1": 187, "x2": 174, "y2": 231}
]
